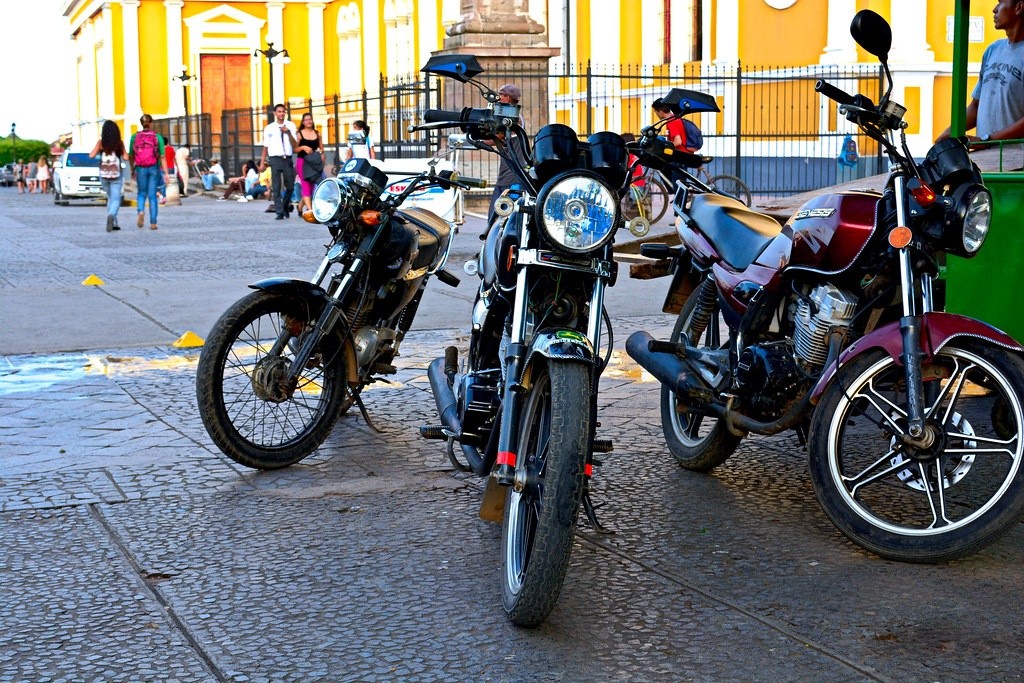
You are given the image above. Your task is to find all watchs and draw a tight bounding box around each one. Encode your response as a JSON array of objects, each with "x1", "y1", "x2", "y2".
[{"x1": 980, "y1": 133, "x2": 991, "y2": 141}]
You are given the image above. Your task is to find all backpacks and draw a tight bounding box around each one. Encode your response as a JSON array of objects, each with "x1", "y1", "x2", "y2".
[
  {"x1": 133, "y1": 130, "x2": 158, "y2": 167},
  {"x1": 679, "y1": 118, "x2": 703, "y2": 151},
  {"x1": 100, "y1": 151, "x2": 121, "y2": 178}
]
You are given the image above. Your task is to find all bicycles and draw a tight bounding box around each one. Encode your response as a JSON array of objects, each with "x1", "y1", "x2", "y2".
[{"x1": 622, "y1": 155, "x2": 752, "y2": 227}]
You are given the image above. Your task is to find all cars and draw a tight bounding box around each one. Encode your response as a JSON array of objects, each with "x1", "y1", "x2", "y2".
[
  {"x1": 52, "y1": 146, "x2": 126, "y2": 208},
  {"x1": 0, "y1": 164, "x2": 17, "y2": 187}
]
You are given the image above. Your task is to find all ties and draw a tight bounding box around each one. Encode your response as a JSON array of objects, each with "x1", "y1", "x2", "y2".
[{"x1": 280, "y1": 126, "x2": 286, "y2": 155}]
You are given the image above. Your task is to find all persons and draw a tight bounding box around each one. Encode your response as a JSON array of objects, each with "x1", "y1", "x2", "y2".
[
  {"x1": 651, "y1": 97, "x2": 694, "y2": 218},
  {"x1": 619, "y1": 132, "x2": 646, "y2": 229},
  {"x1": 281, "y1": 164, "x2": 327, "y2": 202},
  {"x1": 13, "y1": 154, "x2": 51, "y2": 194},
  {"x1": 482, "y1": 83, "x2": 526, "y2": 222},
  {"x1": 199, "y1": 156, "x2": 225, "y2": 191},
  {"x1": 128, "y1": 114, "x2": 171, "y2": 230},
  {"x1": 260, "y1": 103, "x2": 299, "y2": 220},
  {"x1": 935, "y1": 0, "x2": 1024, "y2": 150},
  {"x1": 346, "y1": 120, "x2": 376, "y2": 160},
  {"x1": 293, "y1": 113, "x2": 324, "y2": 218},
  {"x1": 89, "y1": 120, "x2": 129, "y2": 233},
  {"x1": 155, "y1": 136, "x2": 193, "y2": 204},
  {"x1": 218, "y1": 159, "x2": 273, "y2": 202}
]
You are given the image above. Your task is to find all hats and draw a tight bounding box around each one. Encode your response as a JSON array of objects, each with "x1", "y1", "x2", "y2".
[
  {"x1": 209, "y1": 157, "x2": 217, "y2": 163},
  {"x1": 499, "y1": 84, "x2": 521, "y2": 102}
]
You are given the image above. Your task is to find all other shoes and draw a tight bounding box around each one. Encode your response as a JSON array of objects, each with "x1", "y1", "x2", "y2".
[
  {"x1": 282, "y1": 206, "x2": 289, "y2": 218},
  {"x1": 160, "y1": 199, "x2": 167, "y2": 205},
  {"x1": 151, "y1": 225, "x2": 158, "y2": 230},
  {"x1": 297, "y1": 203, "x2": 302, "y2": 218},
  {"x1": 137, "y1": 211, "x2": 145, "y2": 229},
  {"x1": 180, "y1": 193, "x2": 189, "y2": 198},
  {"x1": 237, "y1": 195, "x2": 253, "y2": 203},
  {"x1": 276, "y1": 212, "x2": 281, "y2": 220},
  {"x1": 216, "y1": 196, "x2": 227, "y2": 202},
  {"x1": 107, "y1": 213, "x2": 120, "y2": 231}
]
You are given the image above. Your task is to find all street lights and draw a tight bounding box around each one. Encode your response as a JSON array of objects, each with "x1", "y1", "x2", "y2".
[
  {"x1": 171, "y1": 65, "x2": 197, "y2": 146},
  {"x1": 253, "y1": 32, "x2": 292, "y2": 125},
  {"x1": 10, "y1": 122, "x2": 17, "y2": 163}
]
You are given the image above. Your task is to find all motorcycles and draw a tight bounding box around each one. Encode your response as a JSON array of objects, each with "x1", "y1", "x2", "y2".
[
  {"x1": 625, "y1": 9, "x2": 1024, "y2": 568},
  {"x1": 421, "y1": 54, "x2": 721, "y2": 631},
  {"x1": 196, "y1": 133, "x2": 487, "y2": 471}
]
